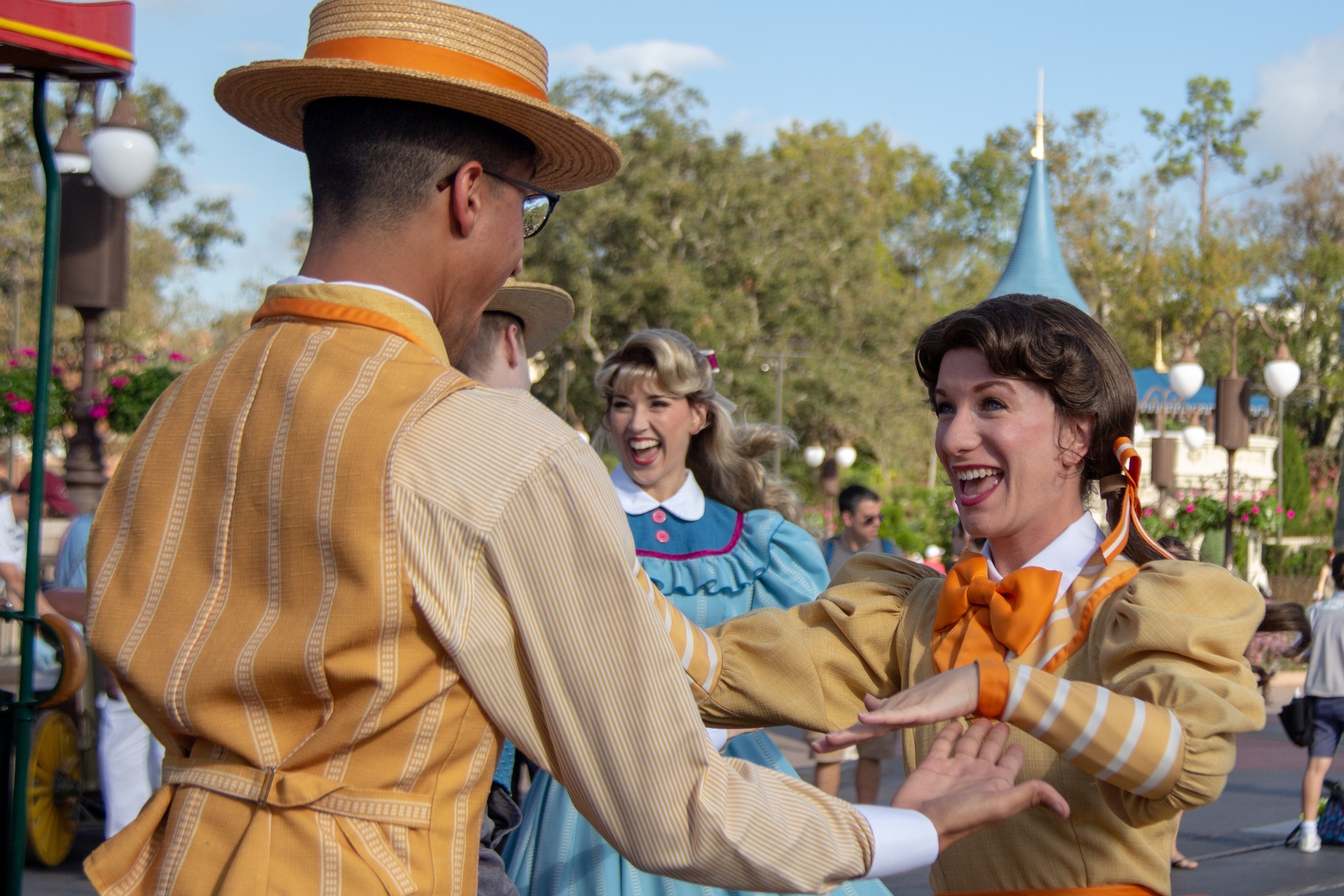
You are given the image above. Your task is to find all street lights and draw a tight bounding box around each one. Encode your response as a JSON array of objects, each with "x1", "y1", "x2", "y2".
[
  {"x1": 51, "y1": 72, "x2": 159, "y2": 518},
  {"x1": 806, "y1": 419, "x2": 860, "y2": 541},
  {"x1": 1168, "y1": 300, "x2": 1305, "y2": 575}
]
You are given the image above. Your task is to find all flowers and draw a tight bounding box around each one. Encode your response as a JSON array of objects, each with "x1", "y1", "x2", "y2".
[
  {"x1": 81, "y1": 351, "x2": 193, "y2": 439},
  {"x1": 1, "y1": 347, "x2": 78, "y2": 454}
]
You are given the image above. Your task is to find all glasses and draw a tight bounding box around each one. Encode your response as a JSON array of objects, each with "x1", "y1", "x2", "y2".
[
  {"x1": 862, "y1": 514, "x2": 884, "y2": 526},
  {"x1": 436, "y1": 166, "x2": 561, "y2": 241}
]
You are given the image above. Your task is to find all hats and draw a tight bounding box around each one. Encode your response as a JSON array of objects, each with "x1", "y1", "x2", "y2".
[
  {"x1": 482, "y1": 277, "x2": 576, "y2": 360},
  {"x1": 18, "y1": 469, "x2": 80, "y2": 516},
  {"x1": 213, "y1": 1, "x2": 625, "y2": 193}
]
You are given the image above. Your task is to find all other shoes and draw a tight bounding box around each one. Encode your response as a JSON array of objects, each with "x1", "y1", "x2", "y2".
[{"x1": 1170, "y1": 857, "x2": 1198, "y2": 870}]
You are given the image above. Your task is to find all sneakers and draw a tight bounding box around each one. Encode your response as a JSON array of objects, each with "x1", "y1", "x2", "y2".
[{"x1": 1299, "y1": 833, "x2": 1322, "y2": 853}]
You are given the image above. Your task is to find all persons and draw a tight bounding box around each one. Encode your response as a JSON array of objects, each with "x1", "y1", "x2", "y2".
[
  {"x1": 1313, "y1": 548, "x2": 1338, "y2": 602},
  {"x1": 79, "y1": 2, "x2": 1079, "y2": 896},
  {"x1": 1296, "y1": 550, "x2": 1344, "y2": 855},
  {"x1": 808, "y1": 483, "x2": 904, "y2": 804},
  {"x1": 49, "y1": 506, "x2": 172, "y2": 846},
  {"x1": 0, "y1": 465, "x2": 65, "y2": 637},
  {"x1": 627, "y1": 290, "x2": 1269, "y2": 896},
  {"x1": 448, "y1": 283, "x2": 581, "y2": 896},
  {"x1": 907, "y1": 518, "x2": 972, "y2": 580},
  {"x1": 492, "y1": 329, "x2": 901, "y2": 895},
  {"x1": 1154, "y1": 534, "x2": 1201, "y2": 870}
]
